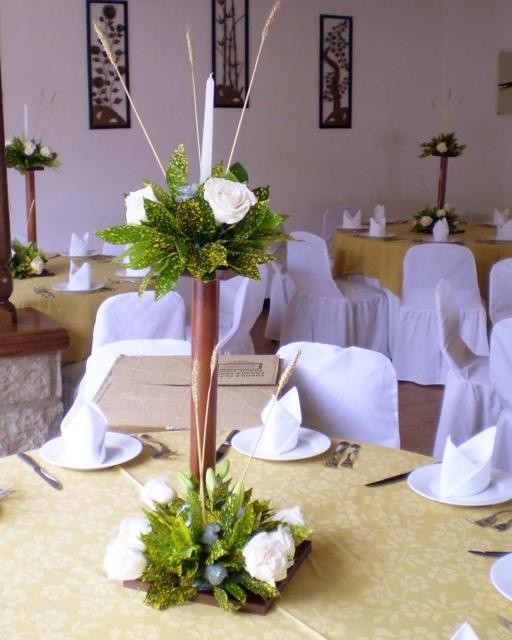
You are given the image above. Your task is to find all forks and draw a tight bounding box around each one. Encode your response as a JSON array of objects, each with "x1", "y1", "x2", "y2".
[
  {"x1": 323, "y1": 441, "x2": 358, "y2": 469},
  {"x1": 465, "y1": 509, "x2": 512, "y2": 533},
  {"x1": 133, "y1": 434, "x2": 174, "y2": 459}
]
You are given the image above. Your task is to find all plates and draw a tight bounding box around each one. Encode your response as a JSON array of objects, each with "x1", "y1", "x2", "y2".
[
  {"x1": 116, "y1": 269, "x2": 148, "y2": 278},
  {"x1": 58, "y1": 251, "x2": 98, "y2": 259},
  {"x1": 407, "y1": 463, "x2": 512, "y2": 506},
  {"x1": 52, "y1": 282, "x2": 103, "y2": 296},
  {"x1": 334, "y1": 217, "x2": 511, "y2": 244},
  {"x1": 39, "y1": 431, "x2": 142, "y2": 470},
  {"x1": 231, "y1": 427, "x2": 330, "y2": 461},
  {"x1": 490, "y1": 553, "x2": 511, "y2": 603}
]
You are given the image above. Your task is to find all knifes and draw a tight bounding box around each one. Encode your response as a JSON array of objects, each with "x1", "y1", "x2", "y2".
[
  {"x1": 17, "y1": 452, "x2": 62, "y2": 490},
  {"x1": 364, "y1": 461, "x2": 441, "y2": 486},
  {"x1": 468, "y1": 545, "x2": 511, "y2": 559},
  {"x1": 216, "y1": 429, "x2": 238, "y2": 460}
]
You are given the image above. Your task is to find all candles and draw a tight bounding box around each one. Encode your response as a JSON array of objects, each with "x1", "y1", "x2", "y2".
[
  {"x1": 23, "y1": 103, "x2": 28, "y2": 139},
  {"x1": 199, "y1": 72, "x2": 215, "y2": 185}
]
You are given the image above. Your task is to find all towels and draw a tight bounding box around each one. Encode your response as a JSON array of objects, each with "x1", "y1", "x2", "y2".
[
  {"x1": 449, "y1": 621, "x2": 482, "y2": 640},
  {"x1": 498, "y1": 222, "x2": 511, "y2": 240},
  {"x1": 494, "y1": 207, "x2": 509, "y2": 225},
  {"x1": 433, "y1": 218, "x2": 449, "y2": 242},
  {"x1": 369, "y1": 204, "x2": 386, "y2": 237},
  {"x1": 440, "y1": 425, "x2": 497, "y2": 497},
  {"x1": 341, "y1": 210, "x2": 362, "y2": 230}
]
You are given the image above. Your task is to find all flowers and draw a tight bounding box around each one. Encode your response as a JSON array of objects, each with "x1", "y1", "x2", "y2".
[
  {"x1": 4, "y1": 88, "x2": 64, "y2": 176},
  {"x1": 95, "y1": 0, "x2": 312, "y2": 300},
  {"x1": 103, "y1": 345, "x2": 316, "y2": 615},
  {"x1": 418, "y1": 96, "x2": 466, "y2": 158},
  {"x1": 10, "y1": 239, "x2": 47, "y2": 279}
]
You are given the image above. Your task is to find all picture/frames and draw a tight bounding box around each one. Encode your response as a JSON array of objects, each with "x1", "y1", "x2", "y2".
[
  {"x1": 211, "y1": 0, "x2": 250, "y2": 108},
  {"x1": 85, "y1": 0, "x2": 130, "y2": 129},
  {"x1": 319, "y1": 14, "x2": 353, "y2": 129}
]
